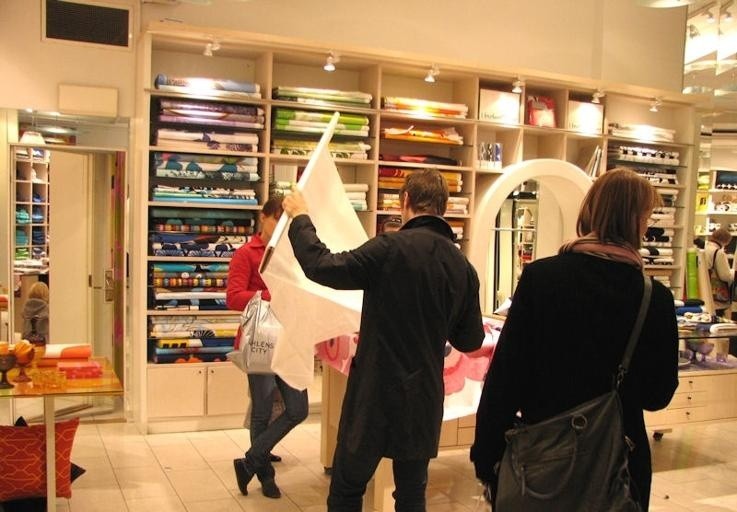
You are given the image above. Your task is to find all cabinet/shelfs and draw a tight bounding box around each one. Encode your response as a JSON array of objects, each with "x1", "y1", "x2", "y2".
[{"x1": 2, "y1": 112, "x2": 119, "y2": 345}]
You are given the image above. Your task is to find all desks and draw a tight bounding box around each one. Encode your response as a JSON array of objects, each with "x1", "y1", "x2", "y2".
[{"x1": 2, "y1": 340, "x2": 123, "y2": 512}]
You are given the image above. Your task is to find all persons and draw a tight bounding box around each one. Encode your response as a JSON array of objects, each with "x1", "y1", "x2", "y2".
[
  {"x1": 701, "y1": 226, "x2": 735, "y2": 317},
  {"x1": 21, "y1": 282, "x2": 49, "y2": 346},
  {"x1": 471, "y1": 167, "x2": 680, "y2": 512},
  {"x1": 226, "y1": 192, "x2": 309, "y2": 499},
  {"x1": 281, "y1": 167, "x2": 486, "y2": 512}
]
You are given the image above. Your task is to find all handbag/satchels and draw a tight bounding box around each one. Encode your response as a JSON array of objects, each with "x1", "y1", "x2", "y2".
[
  {"x1": 225, "y1": 291, "x2": 287, "y2": 375},
  {"x1": 708, "y1": 249, "x2": 731, "y2": 302},
  {"x1": 490, "y1": 388, "x2": 646, "y2": 512}
]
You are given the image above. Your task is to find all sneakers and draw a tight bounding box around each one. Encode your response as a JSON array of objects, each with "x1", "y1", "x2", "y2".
[
  {"x1": 245, "y1": 449, "x2": 282, "y2": 462},
  {"x1": 262, "y1": 479, "x2": 282, "y2": 498},
  {"x1": 233, "y1": 457, "x2": 252, "y2": 496}
]
